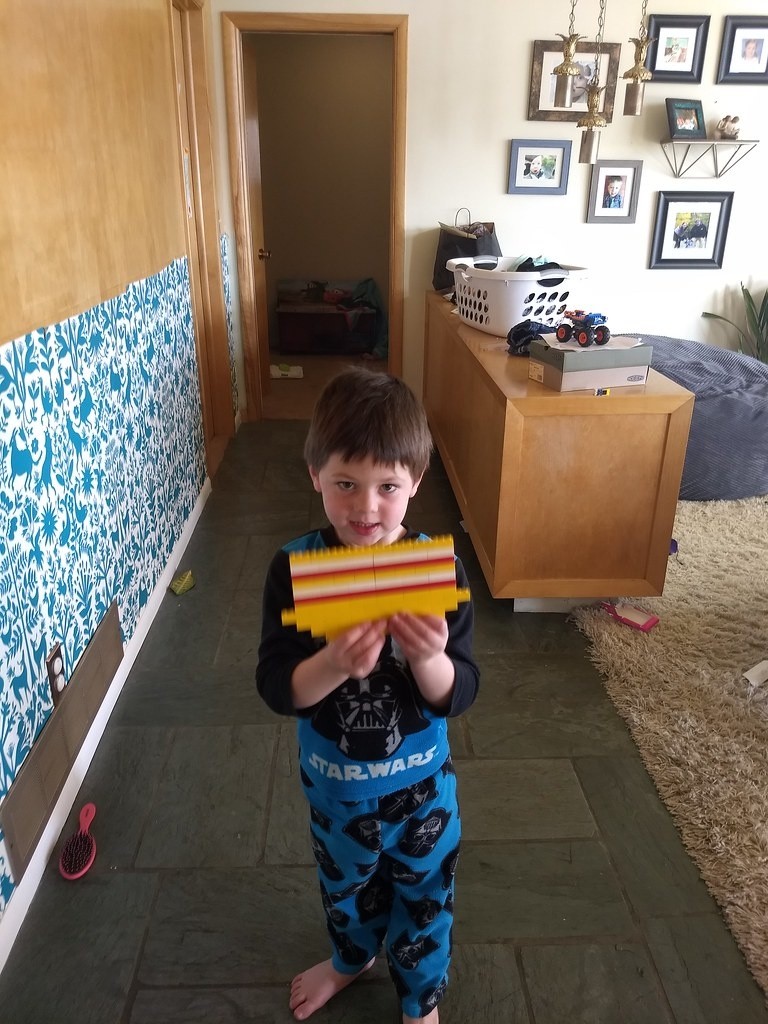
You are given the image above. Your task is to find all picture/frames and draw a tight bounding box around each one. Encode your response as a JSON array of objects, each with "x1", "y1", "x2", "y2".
[
  {"x1": 716, "y1": 15, "x2": 768, "y2": 86},
  {"x1": 528, "y1": 40, "x2": 622, "y2": 124},
  {"x1": 585, "y1": 160, "x2": 644, "y2": 224},
  {"x1": 506, "y1": 139, "x2": 572, "y2": 195},
  {"x1": 644, "y1": 13, "x2": 712, "y2": 84},
  {"x1": 664, "y1": 98, "x2": 707, "y2": 140},
  {"x1": 649, "y1": 191, "x2": 735, "y2": 269}
]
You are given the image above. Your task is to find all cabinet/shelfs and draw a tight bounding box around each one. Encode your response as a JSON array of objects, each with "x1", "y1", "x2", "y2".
[
  {"x1": 421, "y1": 290, "x2": 697, "y2": 614},
  {"x1": 275, "y1": 301, "x2": 376, "y2": 356}
]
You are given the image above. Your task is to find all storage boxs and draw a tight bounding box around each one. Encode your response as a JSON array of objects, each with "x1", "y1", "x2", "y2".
[
  {"x1": 528, "y1": 340, "x2": 654, "y2": 393},
  {"x1": 446, "y1": 255, "x2": 573, "y2": 338}
]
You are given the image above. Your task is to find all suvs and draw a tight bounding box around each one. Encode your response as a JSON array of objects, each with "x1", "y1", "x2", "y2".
[{"x1": 556, "y1": 310, "x2": 612, "y2": 348}]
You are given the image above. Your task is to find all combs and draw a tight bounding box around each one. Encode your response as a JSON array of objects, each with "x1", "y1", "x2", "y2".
[{"x1": 58, "y1": 803, "x2": 97, "y2": 880}]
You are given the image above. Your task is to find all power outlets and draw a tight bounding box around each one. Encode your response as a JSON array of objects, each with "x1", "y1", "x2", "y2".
[{"x1": 45, "y1": 643, "x2": 67, "y2": 707}]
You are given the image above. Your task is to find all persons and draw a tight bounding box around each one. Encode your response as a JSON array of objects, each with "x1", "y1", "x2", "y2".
[
  {"x1": 574, "y1": 61, "x2": 596, "y2": 105},
  {"x1": 742, "y1": 39, "x2": 759, "y2": 64},
  {"x1": 523, "y1": 155, "x2": 545, "y2": 179},
  {"x1": 602, "y1": 176, "x2": 623, "y2": 208},
  {"x1": 717, "y1": 116, "x2": 739, "y2": 135},
  {"x1": 255, "y1": 369, "x2": 480, "y2": 1024},
  {"x1": 674, "y1": 220, "x2": 707, "y2": 248}
]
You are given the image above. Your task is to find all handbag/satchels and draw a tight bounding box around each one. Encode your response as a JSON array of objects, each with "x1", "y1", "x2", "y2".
[{"x1": 432, "y1": 208, "x2": 503, "y2": 291}]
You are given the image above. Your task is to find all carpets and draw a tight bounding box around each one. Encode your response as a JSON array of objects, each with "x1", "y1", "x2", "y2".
[{"x1": 569, "y1": 495, "x2": 768, "y2": 994}]
